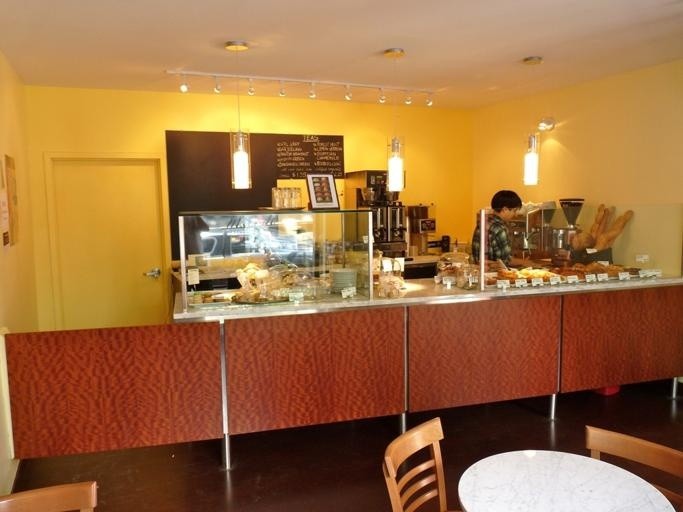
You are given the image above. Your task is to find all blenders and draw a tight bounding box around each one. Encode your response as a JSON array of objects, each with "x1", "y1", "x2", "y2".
[{"x1": 560, "y1": 199, "x2": 584, "y2": 250}]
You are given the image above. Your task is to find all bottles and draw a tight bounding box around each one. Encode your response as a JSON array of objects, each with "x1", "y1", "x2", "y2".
[{"x1": 373, "y1": 251, "x2": 380, "y2": 289}]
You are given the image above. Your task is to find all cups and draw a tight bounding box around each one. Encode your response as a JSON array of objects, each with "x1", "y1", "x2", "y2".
[{"x1": 271, "y1": 187, "x2": 302, "y2": 208}]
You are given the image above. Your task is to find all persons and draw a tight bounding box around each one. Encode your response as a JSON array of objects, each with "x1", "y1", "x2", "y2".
[{"x1": 472, "y1": 190, "x2": 522, "y2": 271}]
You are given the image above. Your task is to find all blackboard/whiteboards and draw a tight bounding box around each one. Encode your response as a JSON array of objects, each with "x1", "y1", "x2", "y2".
[{"x1": 166, "y1": 130, "x2": 344, "y2": 261}]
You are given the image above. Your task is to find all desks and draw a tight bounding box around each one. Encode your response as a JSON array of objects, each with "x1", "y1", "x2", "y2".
[{"x1": 456, "y1": 446, "x2": 676, "y2": 512}]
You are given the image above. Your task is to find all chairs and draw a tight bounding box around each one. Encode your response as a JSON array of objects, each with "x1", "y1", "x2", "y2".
[
  {"x1": 379, "y1": 410, "x2": 447, "y2": 510},
  {"x1": 584, "y1": 424, "x2": 682, "y2": 491}
]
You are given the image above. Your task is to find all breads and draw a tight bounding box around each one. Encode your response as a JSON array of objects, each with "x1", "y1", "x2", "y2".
[{"x1": 488, "y1": 203, "x2": 633, "y2": 285}]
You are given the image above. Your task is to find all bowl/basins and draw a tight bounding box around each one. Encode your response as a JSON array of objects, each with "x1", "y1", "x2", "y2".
[{"x1": 408, "y1": 206, "x2": 428, "y2": 219}]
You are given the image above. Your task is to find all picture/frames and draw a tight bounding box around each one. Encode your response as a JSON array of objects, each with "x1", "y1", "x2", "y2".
[{"x1": 305, "y1": 170, "x2": 340, "y2": 210}]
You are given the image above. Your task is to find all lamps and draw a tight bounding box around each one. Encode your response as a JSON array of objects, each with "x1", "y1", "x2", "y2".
[
  {"x1": 222, "y1": 37, "x2": 253, "y2": 192},
  {"x1": 383, "y1": 46, "x2": 404, "y2": 192},
  {"x1": 522, "y1": 56, "x2": 540, "y2": 187}
]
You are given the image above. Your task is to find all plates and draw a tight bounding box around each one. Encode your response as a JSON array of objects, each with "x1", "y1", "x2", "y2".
[{"x1": 331, "y1": 269, "x2": 356, "y2": 294}]
